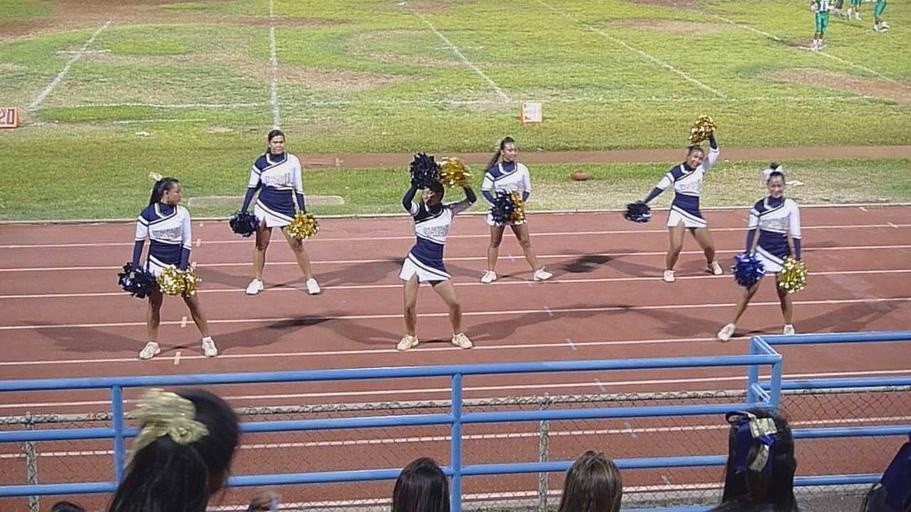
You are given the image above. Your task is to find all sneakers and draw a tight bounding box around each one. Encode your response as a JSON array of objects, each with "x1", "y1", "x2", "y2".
[
  {"x1": 664, "y1": 270, "x2": 676, "y2": 282},
  {"x1": 481, "y1": 270, "x2": 497, "y2": 283},
  {"x1": 452, "y1": 332, "x2": 472, "y2": 349},
  {"x1": 534, "y1": 266, "x2": 553, "y2": 280},
  {"x1": 783, "y1": 326, "x2": 794, "y2": 335},
  {"x1": 397, "y1": 334, "x2": 419, "y2": 351},
  {"x1": 717, "y1": 323, "x2": 736, "y2": 342},
  {"x1": 707, "y1": 261, "x2": 723, "y2": 275},
  {"x1": 202, "y1": 336, "x2": 218, "y2": 357},
  {"x1": 139, "y1": 342, "x2": 161, "y2": 359},
  {"x1": 306, "y1": 278, "x2": 321, "y2": 294},
  {"x1": 246, "y1": 279, "x2": 264, "y2": 295}
]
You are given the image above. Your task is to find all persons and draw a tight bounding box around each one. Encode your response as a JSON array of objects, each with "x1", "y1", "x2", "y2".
[
  {"x1": 558, "y1": 449, "x2": 623, "y2": 511},
  {"x1": 810, "y1": 0, "x2": 887, "y2": 52},
  {"x1": 628, "y1": 119, "x2": 723, "y2": 283},
  {"x1": 397, "y1": 154, "x2": 477, "y2": 352},
  {"x1": 717, "y1": 163, "x2": 806, "y2": 343},
  {"x1": 391, "y1": 456, "x2": 450, "y2": 511},
  {"x1": 53, "y1": 390, "x2": 241, "y2": 512},
  {"x1": 230, "y1": 129, "x2": 320, "y2": 295},
  {"x1": 480, "y1": 137, "x2": 553, "y2": 283},
  {"x1": 859, "y1": 440, "x2": 911, "y2": 512},
  {"x1": 709, "y1": 408, "x2": 799, "y2": 511},
  {"x1": 118, "y1": 177, "x2": 218, "y2": 359}
]
